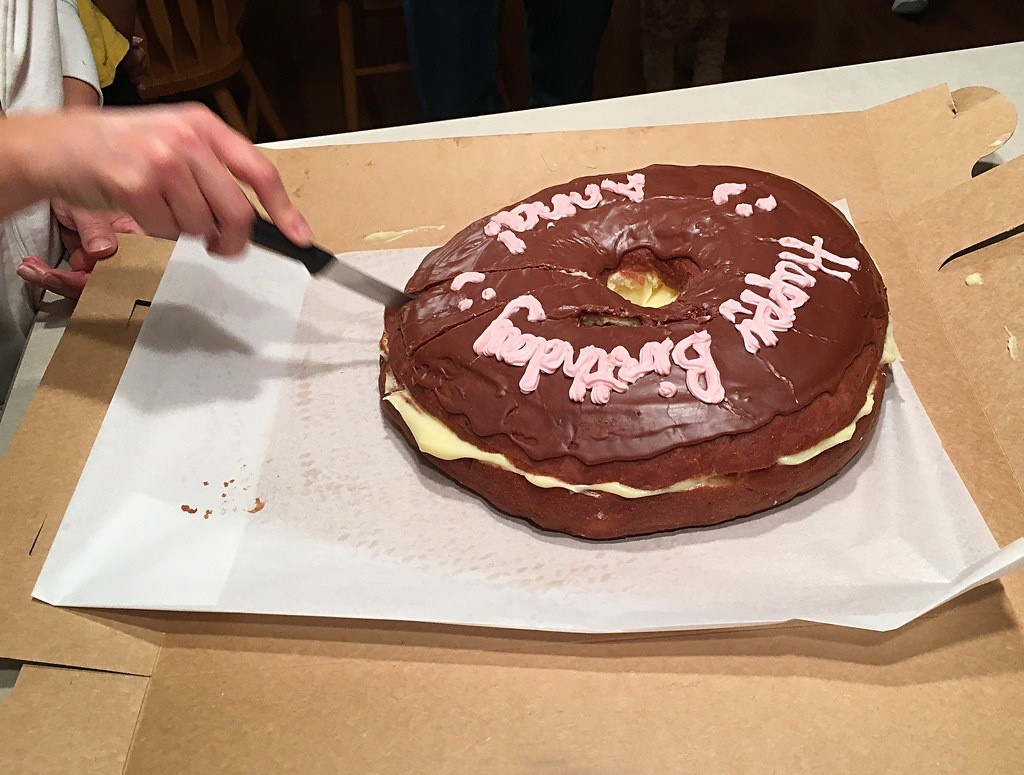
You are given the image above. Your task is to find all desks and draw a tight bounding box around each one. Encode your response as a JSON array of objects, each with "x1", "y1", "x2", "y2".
[{"x1": 0, "y1": 41, "x2": 1024, "y2": 775}]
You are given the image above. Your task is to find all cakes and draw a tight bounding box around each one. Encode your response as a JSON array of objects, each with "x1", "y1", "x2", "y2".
[{"x1": 375, "y1": 162, "x2": 904, "y2": 539}]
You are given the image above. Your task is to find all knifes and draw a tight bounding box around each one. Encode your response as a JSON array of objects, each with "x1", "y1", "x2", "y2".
[{"x1": 247, "y1": 217, "x2": 410, "y2": 313}]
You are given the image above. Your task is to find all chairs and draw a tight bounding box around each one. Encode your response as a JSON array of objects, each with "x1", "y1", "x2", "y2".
[{"x1": 92, "y1": 0, "x2": 289, "y2": 144}]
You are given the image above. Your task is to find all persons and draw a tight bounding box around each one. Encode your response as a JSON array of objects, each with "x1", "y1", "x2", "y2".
[{"x1": 0, "y1": 1, "x2": 316, "y2": 406}]
